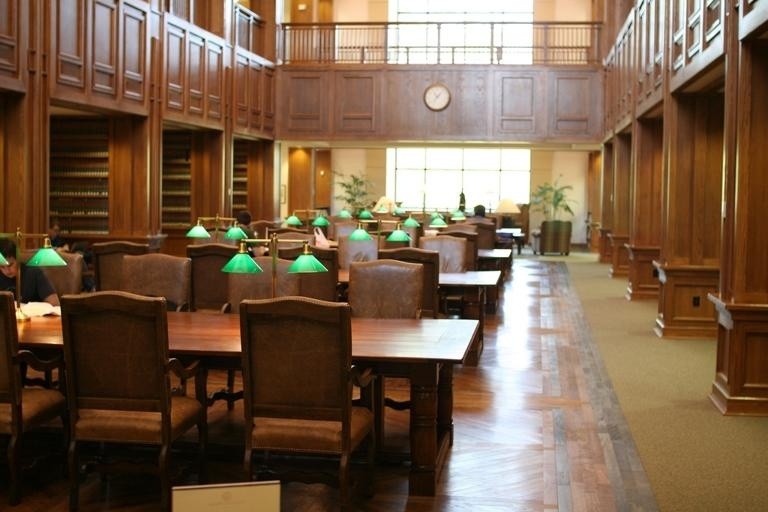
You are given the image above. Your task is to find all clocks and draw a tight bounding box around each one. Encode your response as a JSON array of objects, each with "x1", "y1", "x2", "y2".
[{"x1": 423, "y1": 84, "x2": 451, "y2": 111}]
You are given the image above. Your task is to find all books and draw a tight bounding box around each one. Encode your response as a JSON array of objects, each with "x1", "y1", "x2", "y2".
[
  {"x1": 233, "y1": 142, "x2": 248, "y2": 217},
  {"x1": 50, "y1": 118, "x2": 110, "y2": 233},
  {"x1": 163, "y1": 131, "x2": 190, "y2": 230}
]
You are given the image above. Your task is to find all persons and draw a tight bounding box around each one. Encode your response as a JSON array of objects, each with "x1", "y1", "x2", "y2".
[
  {"x1": 51, "y1": 221, "x2": 75, "y2": 248},
  {"x1": 234, "y1": 213, "x2": 257, "y2": 247},
  {"x1": 0, "y1": 240, "x2": 58, "y2": 307},
  {"x1": 466, "y1": 205, "x2": 497, "y2": 225}
]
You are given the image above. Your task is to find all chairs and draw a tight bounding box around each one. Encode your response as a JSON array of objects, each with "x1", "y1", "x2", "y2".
[
  {"x1": 1, "y1": 206, "x2": 520, "y2": 319},
  {"x1": 1, "y1": 289, "x2": 65, "y2": 511},
  {"x1": 240, "y1": 295, "x2": 389, "y2": 510},
  {"x1": 56, "y1": 289, "x2": 210, "y2": 510},
  {"x1": 530, "y1": 221, "x2": 572, "y2": 256}
]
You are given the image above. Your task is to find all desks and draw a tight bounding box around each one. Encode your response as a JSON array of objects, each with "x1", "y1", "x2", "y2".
[
  {"x1": 499, "y1": 232, "x2": 526, "y2": 255},
  {"x1": 16, "y1": 306, "x2": 482, "y2": 499}
]
